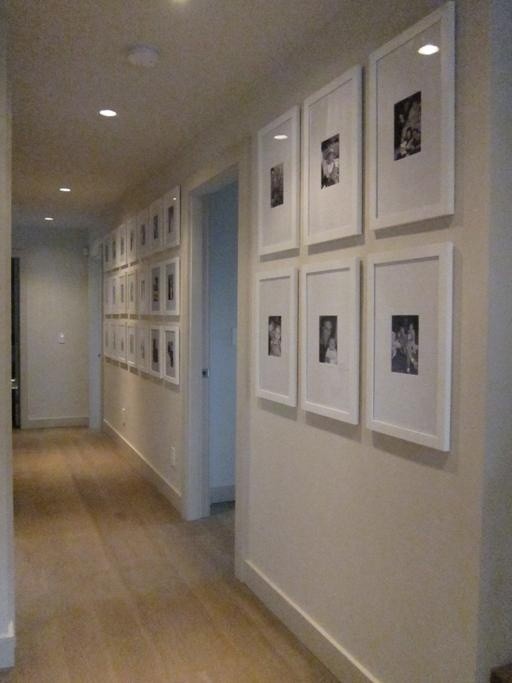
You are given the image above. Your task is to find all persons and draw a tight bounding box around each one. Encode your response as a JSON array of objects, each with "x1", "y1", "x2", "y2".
[
  {"x1": 320, "y1": 320, "x2": 337, "y2": 364},
  {"x1": 320, "y1": 143, "x2": 339, "y2": 185},
  {"x1": 269, "y1": 318, "x2": 281, "y2": 357},
  {"x1": 393, "y1": 322, "x2": 418, "y2": 373},
  {"x1": 396, "y1": 100, "x2": 421, "y2": 159}
]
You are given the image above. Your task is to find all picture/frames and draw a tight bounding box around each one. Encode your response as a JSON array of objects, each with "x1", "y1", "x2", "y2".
[
  {"x1": 301, "y1": 257, "x2": 359, "y2": 426},
  {"x1": 366, "y1": 2, "x2": 455, "y2": 233},
  {"x1": 99, "y1": 185, "x2": 182, "y2": 386},
  {"x1": 250, "y1": 270, "x2": 300, "y2": 407},
  {"x1": 363, "y1": 241, "x2": 453, "y2": 454},
  {"x1": 254, "y1": 106, "x2": 300, "y2": 260},
  {"x1": 303, "y1": 66, "x2": 366, "y2": 248}
]
